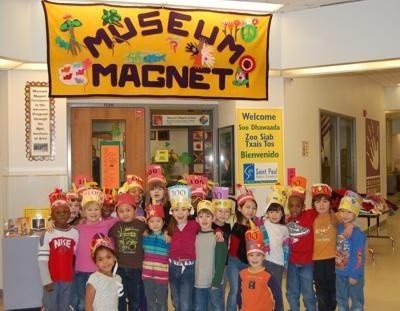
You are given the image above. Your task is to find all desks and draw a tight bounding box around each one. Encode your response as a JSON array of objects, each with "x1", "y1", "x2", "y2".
[{"x1": 358, "y1": 208, "x2": 395, "y2": 254}]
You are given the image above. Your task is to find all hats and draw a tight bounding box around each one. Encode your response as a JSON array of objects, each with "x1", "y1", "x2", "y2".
[
  {"x1": 246, "y1": 220, "x2": 266, "y2": 256},
  {"x1": 49, "y1": 164, "x2": 256, "y2": 223},
  {"x1": 90, "y1": 232, "x2": 114, "y2": 259},
  {"x1": 266, "y1": 176, "x2": 361, "y2": 218}
]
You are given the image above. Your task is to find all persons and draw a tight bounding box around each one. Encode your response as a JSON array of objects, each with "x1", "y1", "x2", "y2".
[{"x1": 38, "y1": 174, "x2": 368, "y2": 310}]
